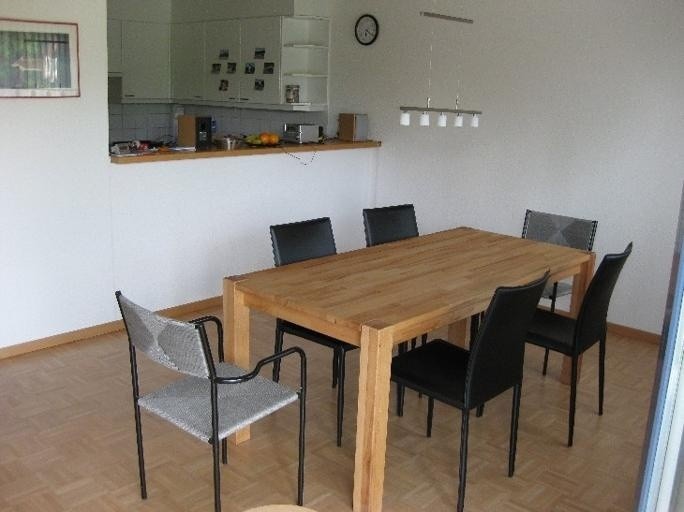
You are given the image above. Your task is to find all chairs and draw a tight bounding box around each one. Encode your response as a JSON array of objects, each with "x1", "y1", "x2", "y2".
[
  {"x1": 517, "y1": 207, "x2": 598, "y2": 378},
  {"x1": 111, "y1": 289, "x2": 310, "y2": 512},
  {"x1": 264, "y1": 215, "x2": 403, "y2": 446},
  {"x1": 388, "y1": 265, "x2": 553, "y2": 511},
  {"x1": 524, "y1": 239, "x2": 634, "y2": 449},
  {"x1": 362, "y1": 206, "x2": 428, "y2": 400}
]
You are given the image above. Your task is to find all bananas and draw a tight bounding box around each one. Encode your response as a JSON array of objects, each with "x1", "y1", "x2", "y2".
[{"x1": 245, "y1": 135, "x2": 261, "y2": 145}]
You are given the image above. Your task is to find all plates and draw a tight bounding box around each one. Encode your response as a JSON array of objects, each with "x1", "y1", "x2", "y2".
[{"x1": 246, "y1": 142, "x2": 282, "y2": 148}]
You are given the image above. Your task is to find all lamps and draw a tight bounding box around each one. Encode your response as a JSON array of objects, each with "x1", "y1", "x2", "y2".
[{"x1": 394, "y1": 2, "x2": 482, "y2": 128}]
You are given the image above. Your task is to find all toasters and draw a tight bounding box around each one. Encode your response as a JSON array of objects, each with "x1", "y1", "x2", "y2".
[{"x1": 278, "y1": 123, "x2": 321, "y2": 145}]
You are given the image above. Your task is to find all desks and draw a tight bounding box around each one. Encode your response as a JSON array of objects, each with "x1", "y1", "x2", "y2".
[{"x1": 224, "y1": 221, "x2": 595, "y2": 511}]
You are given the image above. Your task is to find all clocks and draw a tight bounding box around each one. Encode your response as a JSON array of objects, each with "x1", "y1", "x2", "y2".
[{"x1": 355, "y1": 13, "x2": 379, "y2": 46}]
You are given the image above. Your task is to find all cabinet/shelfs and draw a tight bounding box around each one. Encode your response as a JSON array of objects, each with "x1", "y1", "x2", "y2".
[
  {"x1": 107, "y1": 16, "x2": 122, "y2": 78},
  {"x1": 204, "y1": 16, "x2": 281, "y2": 111},
  {"x1": 168, "y1": 20, "x2": 204, "y2": 104},
  {"x1": 120, "y1": 19, "x2": 171, "y2": 104},
  {"x1": 282, "y1": 14, "x2": 331, "y2": 113}
]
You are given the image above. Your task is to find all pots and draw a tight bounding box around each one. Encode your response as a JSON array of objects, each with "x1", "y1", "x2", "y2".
[{"x1": 215, "y1": 134, "x2": 241, "y2": 151}]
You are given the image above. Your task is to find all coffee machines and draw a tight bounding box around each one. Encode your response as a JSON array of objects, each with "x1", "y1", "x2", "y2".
[{"x1": 177, "y1": 114, "x2": 211, "y2": 151}]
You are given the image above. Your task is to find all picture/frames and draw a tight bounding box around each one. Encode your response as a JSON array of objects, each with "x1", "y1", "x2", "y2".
[{"x1": 0, "y1": 16, "x2": 80, "y2": 99}]
